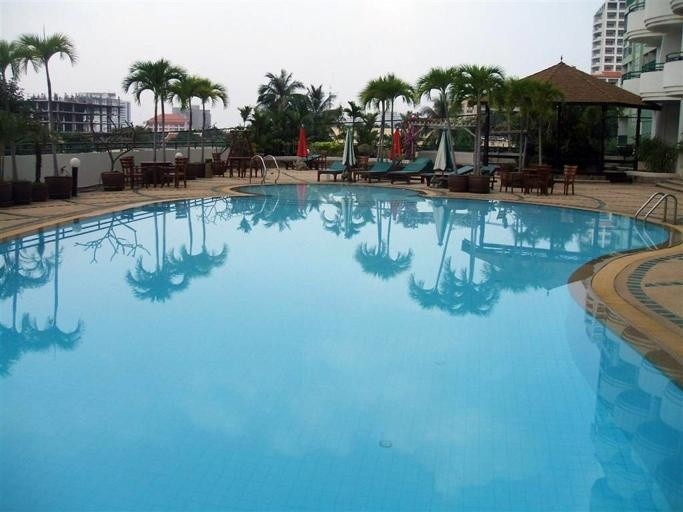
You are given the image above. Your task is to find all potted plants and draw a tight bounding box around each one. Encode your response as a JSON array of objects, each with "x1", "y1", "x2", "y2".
[
  {"x1": 0, "y1": 26, "x2": 71, "y2": 206},
  {"x1": 420, "y1": 67, "x2": 506, "y2": 194},
  {"x1": 89, "y1": 119, "x2": 142, "y2": 191}
]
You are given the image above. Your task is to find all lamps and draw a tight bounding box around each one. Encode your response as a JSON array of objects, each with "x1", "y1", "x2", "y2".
[{"x1": 71, "y1": 158, "x2": 80, "y2": 197}]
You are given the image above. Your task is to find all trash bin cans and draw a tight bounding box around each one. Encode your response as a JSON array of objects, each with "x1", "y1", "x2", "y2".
[{"x1": 205, "y1": 159, "x2": 212, "y2": 178}]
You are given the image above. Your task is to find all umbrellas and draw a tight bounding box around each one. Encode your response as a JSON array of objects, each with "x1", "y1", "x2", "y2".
[
  {"x1": 296, "y1": 184, "x2": 306, "y2": 213},
  {"x1": 295, "y1": 124, "x2": 309, "y2": 159},
  {"x1": 341, "y1": 126, "x2": 358, "y2": 168},
  {"x1": 385, "y1": 126, "x2": 401, "y2": 160},
  {"x1": 340, "y1": 193, "x2": 354, "y2": 236},
  {"x1": 432, "y1": 119, "x2": 456, "y2": 173},
  {"x1": 432, "y1": 204, "x2": 451, "y2": 247}
]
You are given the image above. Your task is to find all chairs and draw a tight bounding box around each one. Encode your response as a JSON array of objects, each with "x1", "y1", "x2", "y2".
[
  {"x1": 284, "y1": 151, "x2": 578, "y2": 195},
  {"x1": 213, "y1": 152, "x2": 263, "y2": 178},
  {"x1": 119, "y1": 155, "x2": 189, "y2": 188}
]
